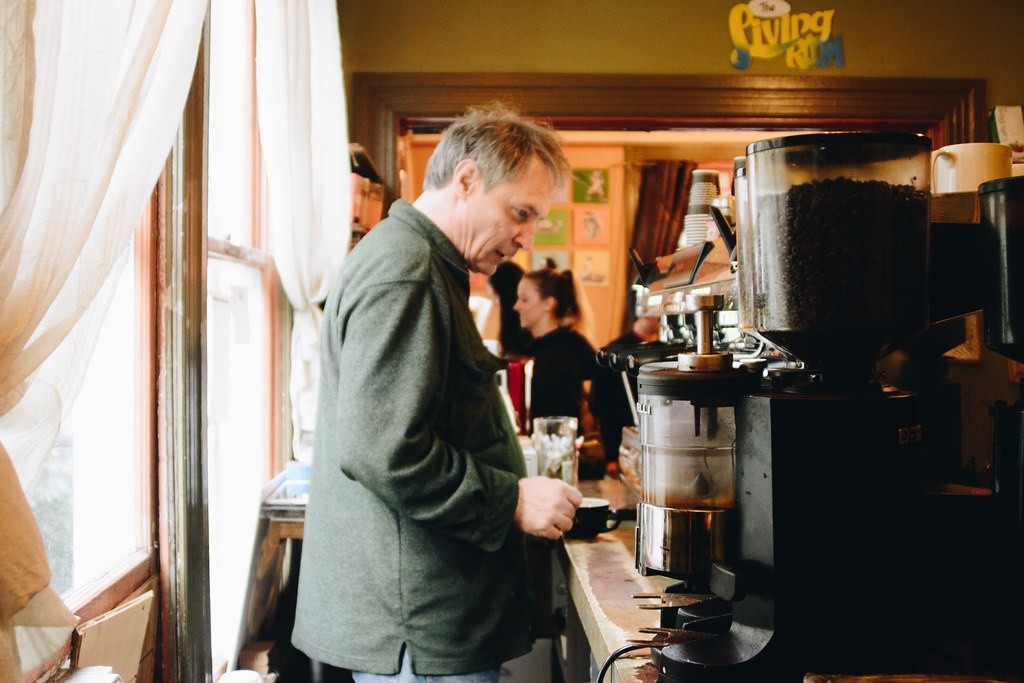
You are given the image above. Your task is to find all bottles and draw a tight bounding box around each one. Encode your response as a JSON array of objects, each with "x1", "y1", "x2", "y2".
[{"x1": 533, "y1": 415, "x2": 579, "y2": 489}]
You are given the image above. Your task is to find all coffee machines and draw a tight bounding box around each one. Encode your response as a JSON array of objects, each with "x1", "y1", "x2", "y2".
[{"x1": 632, "y1": 128, "x2": 932, "y2": 682}]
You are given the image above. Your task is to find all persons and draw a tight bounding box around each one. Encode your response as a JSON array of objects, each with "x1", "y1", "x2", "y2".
[
  {"x1": 487, "y1": 262, "x2": 532, "y2": 355},
  {"x1": 514, "y1": 268, "x2": 599, "y2": 477},
  {"x1": 289, "y1": 99, "x2": 625, "y2": 683}
]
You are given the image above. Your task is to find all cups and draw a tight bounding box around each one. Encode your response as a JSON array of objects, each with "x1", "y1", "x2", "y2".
[
  {"x1": 564, "y1": 497, "x2": 622, "y2": 541},
  {"x1": 931, "y1": 141, "x2": 1012, "y2": 194},
  {"x1": 684, "y1": 157, "x2": 720, "y2": 249}
]
